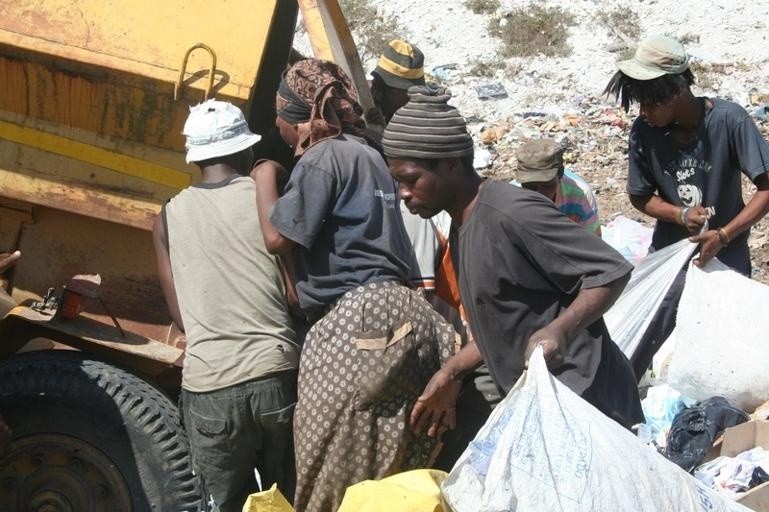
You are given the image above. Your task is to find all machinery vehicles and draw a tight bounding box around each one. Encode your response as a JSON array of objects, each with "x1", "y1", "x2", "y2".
[{"x1": 1, "y1": 2, "x2": 390, "y2": 509}]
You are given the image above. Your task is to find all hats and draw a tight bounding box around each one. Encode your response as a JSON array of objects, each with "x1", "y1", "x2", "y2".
[
  {"x1": 181, "y1": 97, "x2": 263, "y2": 165},
  {"x1": 615, "y1": 36, "x2": 691, "y2": 81},
  {"x1": 515, "y1": 139, "x2": 565, "y2": 184},
  {"x1": 380, "y1": 85, "x2": 474, "y2": 160},
  {"x1": 370, "y1": 40, "x2": 426, "y2": 90}
]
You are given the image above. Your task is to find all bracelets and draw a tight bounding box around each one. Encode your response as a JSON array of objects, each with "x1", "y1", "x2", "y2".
[
  {"x1": 717, "y1": 227, "x2": 730, "y2": 248},
  {"x1": 680, "y1": 206, "x2": 687, "y2": 225}
]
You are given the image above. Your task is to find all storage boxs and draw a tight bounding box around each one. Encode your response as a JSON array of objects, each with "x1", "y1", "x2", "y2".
[{"x1": 637, "y1": 378, "x2": 769, "y2": 512}]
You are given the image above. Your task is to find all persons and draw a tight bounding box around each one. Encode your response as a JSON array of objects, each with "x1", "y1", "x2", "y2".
[
  {"x1": 513, "y1": 135, "x2": 603, "y2": 241},
  {"x1": 370, "y1": 40, "x2": 456, "y2": 323},
  {"x1": 600, "y1": 33, "x2": 769, "y2": 278},
  {"x1": 154, "y1": 98, "x2": 306, "y2": 512},
  {"x1": 252, "y1": 59, "x2": 465, "y2": 512},
  {"x1": 380, "y1": 81, "x2": 644, "y2": 429}
]
